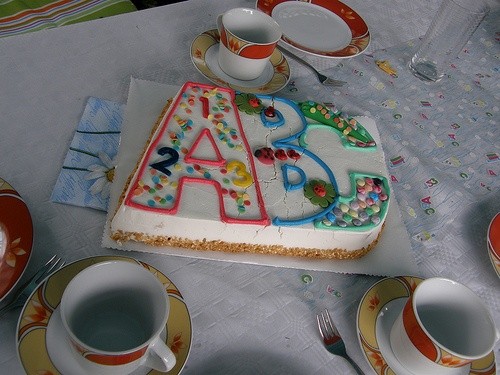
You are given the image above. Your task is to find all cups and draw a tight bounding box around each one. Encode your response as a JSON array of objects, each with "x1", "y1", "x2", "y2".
[
  {"x1": 215, "y1": 7, "x2": 282, "y2": 82},
  {"x1": 409, "y1": 0, "x2": 490, "y2": 83},
  {"x1": 60, "y1": 259, "x2": 176, "y2": 375},
  {"x1": 387, "y1": 277, "x2": 500, "y2": 375}
]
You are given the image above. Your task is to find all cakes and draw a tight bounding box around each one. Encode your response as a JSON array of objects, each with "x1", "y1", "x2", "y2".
[{"x1": 109, "y1": 81, "x2": 391, "y2": 261}]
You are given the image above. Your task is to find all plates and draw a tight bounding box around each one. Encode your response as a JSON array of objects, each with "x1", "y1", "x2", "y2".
[
  {"x1": 486, "y1": 211, "x2": 500, "y2": 278},
  {"x1": 0, "y1": 177, "x2": 34, "y2": 306},
  {"x1": 354, "y1": 276, "x2": 498, "y2": 375},
  {"x1": 15, "y1": 255, "x2": 193, "y2": 375},
  {"x1": 254, "y1": 0, "x2": 371, "y2": 59},
  {"x1": 189, "y1": 29, "x2": 292, "y2": 94}
]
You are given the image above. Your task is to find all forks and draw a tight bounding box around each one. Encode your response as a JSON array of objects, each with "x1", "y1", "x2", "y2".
[
  {"x1": 274, "y1": 44, "x2": 348, "y2": 86},
  {"x1": 0, "y1": 255, "x2": 65, "y2": 321},
  {"x1": 316, "y1": 308, "x2": 367, "y2": 375}
]
